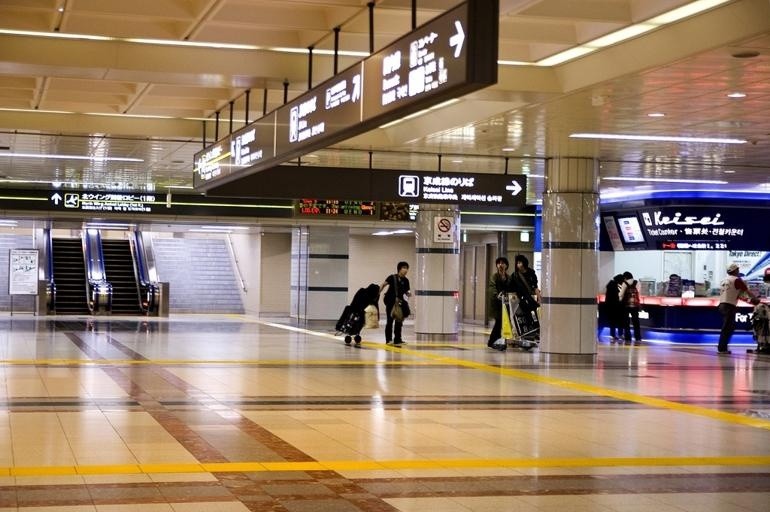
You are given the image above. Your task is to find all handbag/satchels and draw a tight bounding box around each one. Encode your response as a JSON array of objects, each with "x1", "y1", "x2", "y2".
[
  {"x1": 364, "y1": 305, "x2": 380, "y2": 328},
  {"x1": 390, "y1": 297, "x2": 404, "y2": 322},
  {"x1": 350, "y1": 284, "x2": 379, "y2": 314}
]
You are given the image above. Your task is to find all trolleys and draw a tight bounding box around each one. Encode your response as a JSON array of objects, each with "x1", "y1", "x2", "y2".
[{"x1": 494, "y1": 292, "x2": 540, "y2": 351}]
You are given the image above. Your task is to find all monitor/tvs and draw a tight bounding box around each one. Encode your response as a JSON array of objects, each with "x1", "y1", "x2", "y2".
[{"x1": 617, "y1": 213, "x2": 647, "y2": 246}]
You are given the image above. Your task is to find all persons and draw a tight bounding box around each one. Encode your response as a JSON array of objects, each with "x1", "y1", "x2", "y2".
[
  {"x1": 716, "y1": 264, "x2": 757, "y2": 354},
  {"x1": 509, "y1": 254, "x2": 540, "y2": 343},
  {"x1": 597, "y1": 271, "x2": 642, "y2": 346},
  {"x1": 378, "y1": 262, "x2": 412, "y2": 345},
  {"x1": 488, "y1": 257, "x2": 513, "y2": 350}
]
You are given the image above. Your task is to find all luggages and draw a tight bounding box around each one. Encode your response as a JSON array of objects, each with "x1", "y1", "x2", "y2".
[{"x1": 336, "y1": 295, "x2": 379, "y2": 344}]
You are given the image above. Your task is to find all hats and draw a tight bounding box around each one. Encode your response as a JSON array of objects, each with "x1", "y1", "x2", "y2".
[{"x1": 726, "y1": 264, "x2": 739, "y2": 273}]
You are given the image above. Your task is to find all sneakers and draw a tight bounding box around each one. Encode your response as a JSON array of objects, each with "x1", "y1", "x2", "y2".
[
  {"x1": 387, "y1": 341, "x2": 394, "y2": 345},
  {"x1": 393, "y1": 342, "x2": 407, "y2": 346},
  {"x1": 717, "y1": 350, "x2": 731, "y2": 354},
  {"x1": 609, "y1": 335, "x2": 642, "y2": 346}
]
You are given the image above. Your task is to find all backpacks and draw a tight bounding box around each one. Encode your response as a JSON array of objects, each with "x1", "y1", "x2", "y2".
[{"x1": 623, "y1": 280, "x2": 640, "y2": 305}]
